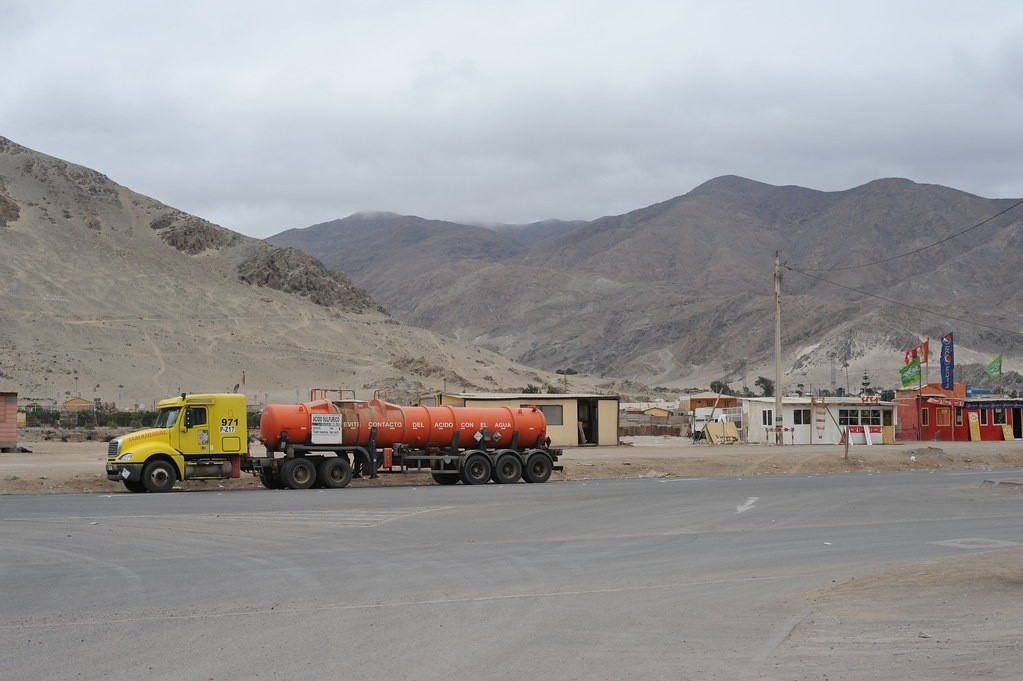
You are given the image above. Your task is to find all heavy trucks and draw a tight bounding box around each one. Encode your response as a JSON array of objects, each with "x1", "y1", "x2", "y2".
[{"x1": 106, "y1": 385, "x2": 563, "y2": 492}]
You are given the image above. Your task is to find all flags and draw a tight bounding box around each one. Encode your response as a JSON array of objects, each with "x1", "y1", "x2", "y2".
[
  {"x1": 984, "y1": 353, "x2": 1002, "y2": 381},
  {"x1": 904, "y1": 340, "x2": 927, "y2": 365},
  {"x1": 899, "y1": 356, "x2": 921, "y2": 387},
  {"x1": 940, "y1": 332, "x2": 954, "y2": 390}
]
